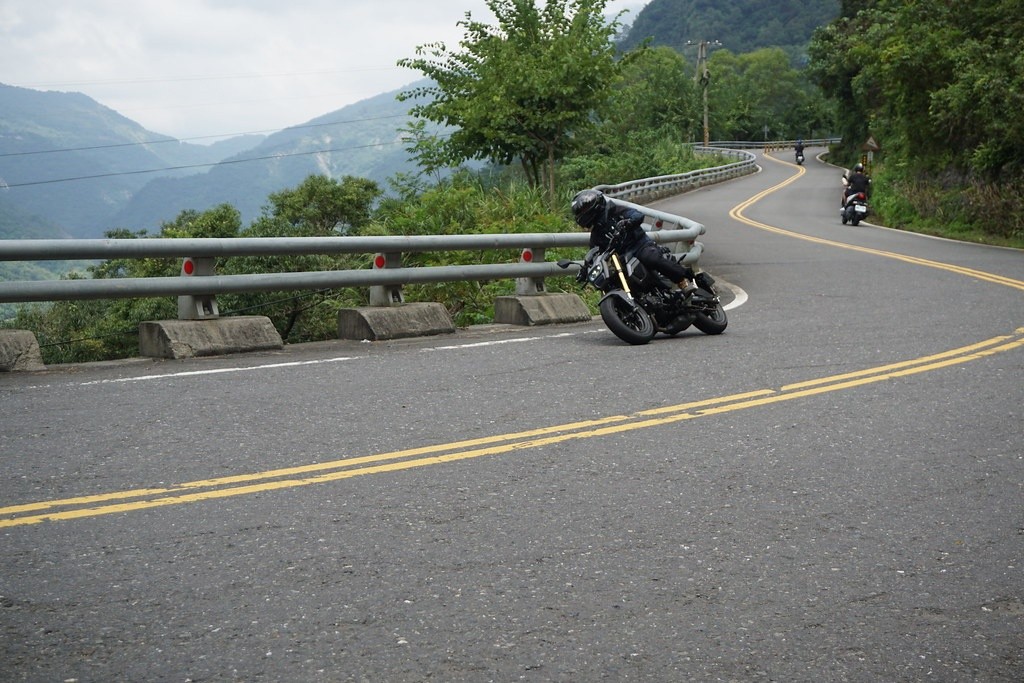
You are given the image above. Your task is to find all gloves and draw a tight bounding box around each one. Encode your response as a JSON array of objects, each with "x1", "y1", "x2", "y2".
[{"x1": 616, "y1": 219, "x2": 631, "y2": 231}]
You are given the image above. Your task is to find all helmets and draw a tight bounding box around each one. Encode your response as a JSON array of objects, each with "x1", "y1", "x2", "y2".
[
  {"x1": 855, "y1": 163, "x2": 863, "y2": 171},
  {"x1": 571, "y1": 189, "x2": 606, "y2": 228}
]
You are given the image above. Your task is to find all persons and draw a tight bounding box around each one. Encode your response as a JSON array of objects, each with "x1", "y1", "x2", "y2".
[
  {"x1": 795, "y1": 141, "x2": 804, "y2": 161},
  {"x1": 570, "y1": 189, "x2": 699, "y2": 300},
  {"x1": 840, "y1": 163, "x2": 872, "y2": 211}
]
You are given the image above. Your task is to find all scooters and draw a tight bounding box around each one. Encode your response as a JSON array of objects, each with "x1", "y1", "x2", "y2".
[
  {"x1": 841, "y1": 174, "x2": 869, "y2": 226},
  {"x1": 796, "y1": 152, "x2": 804, "y2": 165}
]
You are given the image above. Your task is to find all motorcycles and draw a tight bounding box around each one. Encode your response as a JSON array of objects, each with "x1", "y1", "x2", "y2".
[{"x1": 556, "y1": 223, "x2": 728, "y2": 344}]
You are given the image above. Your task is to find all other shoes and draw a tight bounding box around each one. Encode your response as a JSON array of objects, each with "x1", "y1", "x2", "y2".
[
  {"x1": 840, "y1": 207, "x2": 845, "y2": 210},
  {"x1": 679, "y1": 279, "x2": 697, "y2": 297}
]
